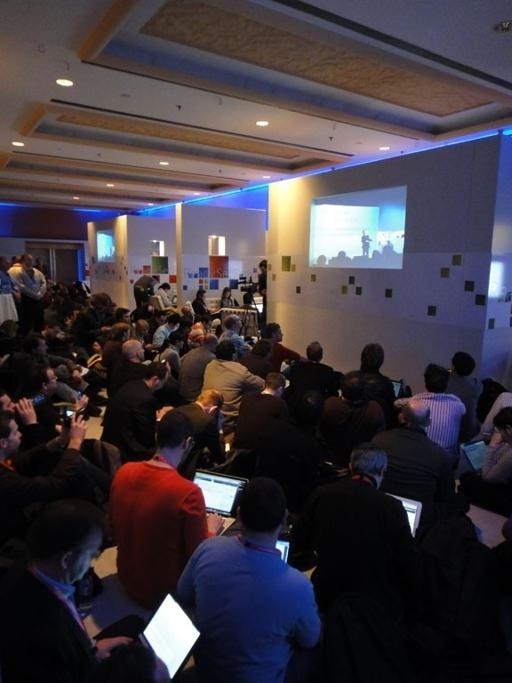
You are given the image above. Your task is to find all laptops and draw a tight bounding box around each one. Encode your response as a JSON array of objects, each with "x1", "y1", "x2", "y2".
[
  {"x1": 189, "y1": 468, "x2": 250, "y2": 543},
  {"x1": 460, "y1": 438, "x2": 489, "y2": 473},
  {"x1": 275, "y1": 540, "x2": 290, "y2": 566},
  {"x1": 390, "y1": 379, "x2": 402, "y2": 399},
  {"x1": 111, "y1": 590, "x2": 204, "y2": 683},
  {"x1": 384, "y1": 490, "x2": 424, "y2": 539}
]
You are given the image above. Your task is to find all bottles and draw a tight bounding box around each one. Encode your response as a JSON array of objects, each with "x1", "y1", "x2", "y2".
[{"x1": 31, "y1": 393, "x2": 47, "y2": 405}]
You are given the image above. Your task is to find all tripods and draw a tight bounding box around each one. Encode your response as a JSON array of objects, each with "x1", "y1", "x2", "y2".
[{"x1": 237, "y1": 294, "x2": 261, "y2": 341}]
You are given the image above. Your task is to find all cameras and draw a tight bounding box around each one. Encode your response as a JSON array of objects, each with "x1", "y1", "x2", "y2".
[{"x1": 63, "y1": 406, "x2": 91, "y2": 425}]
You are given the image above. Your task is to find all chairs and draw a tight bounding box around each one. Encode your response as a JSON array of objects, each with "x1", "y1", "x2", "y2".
[{"x1": 147, "y1": 292, "x2": 264, "y2": 338}]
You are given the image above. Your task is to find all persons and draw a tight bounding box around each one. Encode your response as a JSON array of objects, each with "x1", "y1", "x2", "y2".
[
  {"x1": 312, "y1": 230, "x2": 403, "y2": 268},
  {"x1": 0, "y1": 252, "x2": 511, "y2": 681}
]
